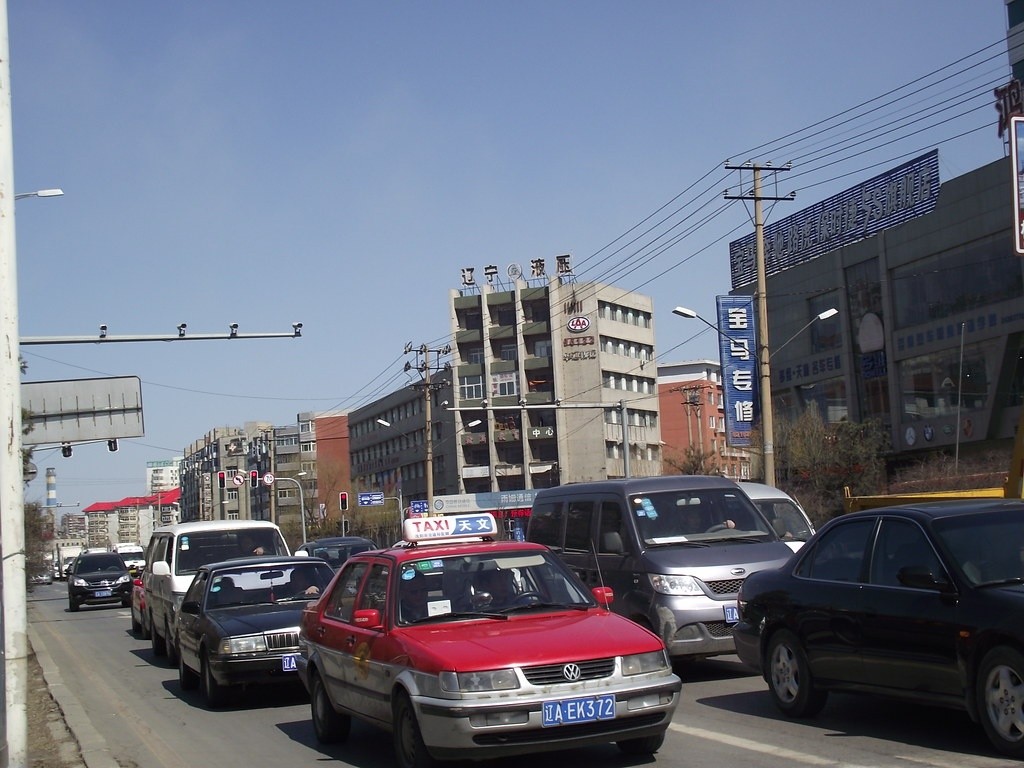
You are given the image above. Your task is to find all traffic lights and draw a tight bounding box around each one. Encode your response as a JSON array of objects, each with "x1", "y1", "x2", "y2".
[
  {"x1": 217, "y1": 471, "x2": 227, "y2": 490},
  {"x1": 344, "y1": 518, "x2": 350, "y2": 533},
  {"x1": 250, "y1": 470, "x2": 258, "y2": 489},
  {"x1": 339, "y1": 491, "x2": 348, "y2": 511}
]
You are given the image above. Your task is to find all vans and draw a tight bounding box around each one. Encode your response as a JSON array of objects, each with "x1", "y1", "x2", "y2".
[
  {"x1": 144, "y1": 519, "x2": 293, "y2": 664},
  {"x1": 677, "y1": 482, "x2": 818, "y2": 555},
  {"x1": 523, "y1": 475, "x2": 797, "y2": 658}
]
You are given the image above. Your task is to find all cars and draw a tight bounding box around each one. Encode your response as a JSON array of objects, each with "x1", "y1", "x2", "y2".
[
  {"x1": 291, "y1": 511, "x2": 683, "y2": 768},
  {"x1": 382, "y1": 536, "x2": 484, "y2": 576},
  {"x1": 170, "y1": 555, "x2": 355, "y2": 707},
  {"x1": 730, "y1": 499, "x2": 1024, "y2": 763},
  {"x1": 129, "y1": 568, "x2": 151, "y2": 641},
  {"x1": 295, "y1": 536, "x2": 380, "y2": 592}
]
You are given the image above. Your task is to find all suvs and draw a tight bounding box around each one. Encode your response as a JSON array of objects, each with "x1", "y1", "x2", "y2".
[{"x1": 66, "y1": 550, "x2": 133, "y2": 612}]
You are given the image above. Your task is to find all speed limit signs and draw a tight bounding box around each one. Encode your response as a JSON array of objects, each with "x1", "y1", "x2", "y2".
[{"x1": 262, "y1": 472, "x2": 276, "y2": 486}]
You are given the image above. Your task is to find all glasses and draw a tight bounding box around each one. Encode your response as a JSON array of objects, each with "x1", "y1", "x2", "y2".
[{"x1": 403, "y1": 585, "x2": 428, "y2": 595}]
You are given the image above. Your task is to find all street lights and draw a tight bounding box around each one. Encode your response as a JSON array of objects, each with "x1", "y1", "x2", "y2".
[
  {"x1": 199, "y1": 500, "x2": 230, "y2": 522},
  {"x1": 658, "y1": 440, "x2": 694, "y2": 474},
  {"x1": 140, "y1": 512, "x2": 180, "y2": 528},
  {"x1": 670, "y1": 305, "x2": 842, "y2": 489},
  {"x1": 237, "y1": 468, "x2": 308, "y2": 525},
  {"x1": 378, "y1": 418, "x2": 482, "y2": 517}
]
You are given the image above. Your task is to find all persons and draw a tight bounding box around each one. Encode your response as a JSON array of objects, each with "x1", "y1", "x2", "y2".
[
  {"x1": 239, "y1": 536, "x2": 268, "y2": 555},
  {"x1": 290, "y1": 570, "x2": 319, "y2": 594},
  {"x1": 762, "y1": 505, "x2": 792, "y2": 537},
  {"x1": 679, "y1": 509, "x2": 736, "y2": 533},
  {"x1": 480, "y1": 570, "x2": 529, "y2": 606},
  {"x1": 401, "y1": 571, "x2": 432, "y2": 620}
]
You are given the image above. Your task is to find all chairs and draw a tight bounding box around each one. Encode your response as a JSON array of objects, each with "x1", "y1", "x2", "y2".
[{"x1": 213, "y1": 576, "x2": 245, "y2": 604}]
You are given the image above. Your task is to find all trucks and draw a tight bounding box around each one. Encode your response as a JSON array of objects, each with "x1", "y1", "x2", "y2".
[{"x1": 28, "y1": 544, "x2": 147, "y2": 585}]
